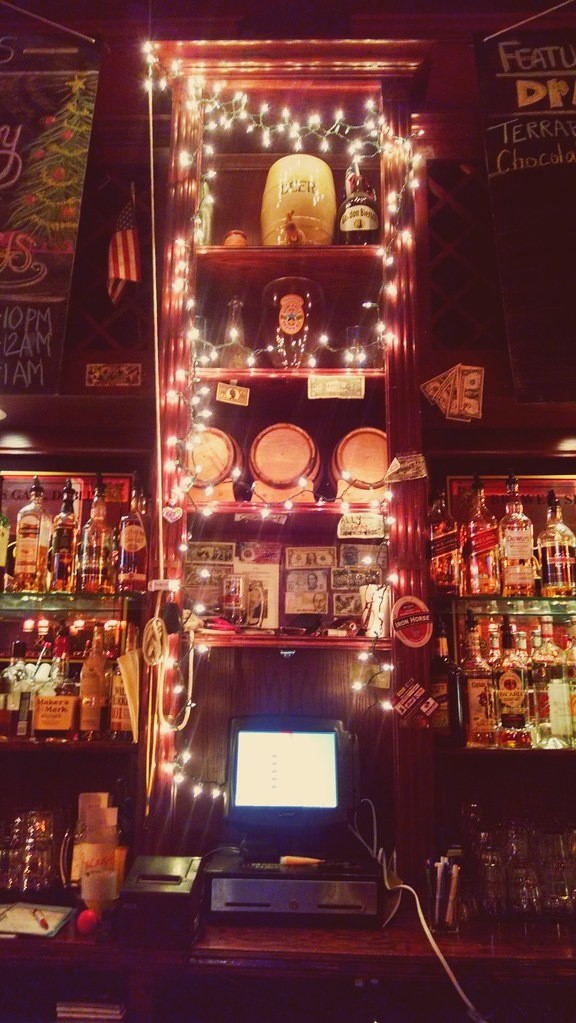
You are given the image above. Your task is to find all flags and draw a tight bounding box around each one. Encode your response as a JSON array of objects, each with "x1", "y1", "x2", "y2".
[{"x1": 106, "y1": 199, "x2": 142, "y2": 307}]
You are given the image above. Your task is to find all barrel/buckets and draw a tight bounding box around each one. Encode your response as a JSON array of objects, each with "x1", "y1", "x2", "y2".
[{"x1": 183, "y1": 425, "x2": 390, "y2": 491}]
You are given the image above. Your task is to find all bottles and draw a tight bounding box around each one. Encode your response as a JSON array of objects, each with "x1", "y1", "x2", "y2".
[
  {"x1": 0, "y1": 470, "x2": 150, "y2": 596},
  {"x1": 221, "y1": 296, "x2": 248, "y2": 369},
  {"x1": 0, "y1": 621, "x2": 138, "y2": 742},
  {"x1": 73, "y1": 791, "x2": 117, "y2": 898},
  {"x1": 432, "y1": 608, "x2": 576, "y2": 750},
  {"x1": 339, "y1": 165, "x2": 380, "y2": 245},
  {"x1": 0, "y1": 808, "x2": 121, "y2": 893},
  {"x1": 429, "y1": 474, "x2": 576, "y2": 598}
]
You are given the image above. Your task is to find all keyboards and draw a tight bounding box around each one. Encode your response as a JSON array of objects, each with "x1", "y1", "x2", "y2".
[{"x1": 240, "y1": 857, "x2": 370, "y2": 874}]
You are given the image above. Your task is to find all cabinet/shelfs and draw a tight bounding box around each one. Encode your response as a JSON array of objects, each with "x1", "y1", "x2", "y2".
[
  {"x1": 433, "y1": 455, "x2": 576, "y2": 829},
  {"x1": 0, "y1": 471, "x2": 157, "y2": 911},
  {"x1": 127, "y1": 38, "x2": 442, "y2": 924}
]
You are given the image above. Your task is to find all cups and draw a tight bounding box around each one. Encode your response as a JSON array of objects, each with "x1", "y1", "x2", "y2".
[
  {"x1": 261, "y1": 274, "x2": 321, "y2": 368},
  {"x1": 193, "y1": 315, "x2": 214, "y2": 368},
  {"x1": 221, "y1": 574, "x2": 265, "y2": 630},
  {"x1": 359, "y1": 585, "x2": 391, "y2": 638},
  {"x1": 344, "y1": 326, "x2": 369, "y2": 368},
  {"x1": 427, "y1": 800, "x2": 576, "y2": 934}
]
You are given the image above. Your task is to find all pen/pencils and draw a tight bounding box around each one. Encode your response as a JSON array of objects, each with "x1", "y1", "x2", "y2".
[{"x1": 31, "y1": 908, "x2": 48, "y2": 930}]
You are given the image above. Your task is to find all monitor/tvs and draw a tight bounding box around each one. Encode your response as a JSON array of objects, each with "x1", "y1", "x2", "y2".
[{"x1": 222, "y1": 715, "x2": 359, "y2": 829}]
